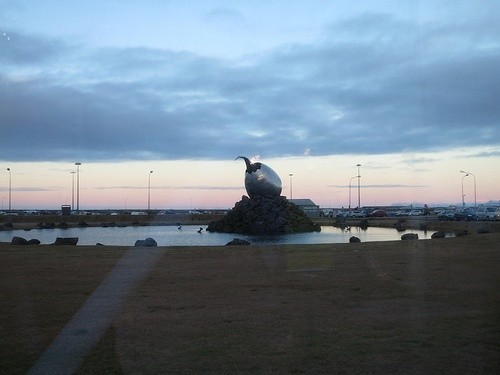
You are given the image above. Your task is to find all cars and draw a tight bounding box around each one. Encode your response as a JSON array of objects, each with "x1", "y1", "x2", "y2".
[
  {"x1": 319, "y1": 210, "x2": 422, "y2": 218},
  {"x1": 434, "y1": 206, "x2": 499, "y2": 221}
]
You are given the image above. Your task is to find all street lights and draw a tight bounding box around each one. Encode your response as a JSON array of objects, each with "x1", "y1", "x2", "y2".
[
  {"x1": 148, "y1": 171, "x2": 153, "y2": 209},
  {"x1": 349, "y1": 175, "x2": 360, "y2": 207},
  {"x1": 71, "y1": 171, "x2": 75, "y2": 209},
  {"x1": 356, "y1": 164, "x2": 361, "y2": 208},
  {"x1": 460, "y1": 170, "x2": 476, "y2": 214},
  {"x1": 75, "y1": 162, "x2": 81, "y2": 210},
  {"x1": 461, "y1": 173, "x2": 469, "y2": 208},
  {"x1": 7, "y1": 168, "x2": 10, "y2": 215},
  {"x1": 288, "y1": 173, "x2": 294, "y2": 199}
]
